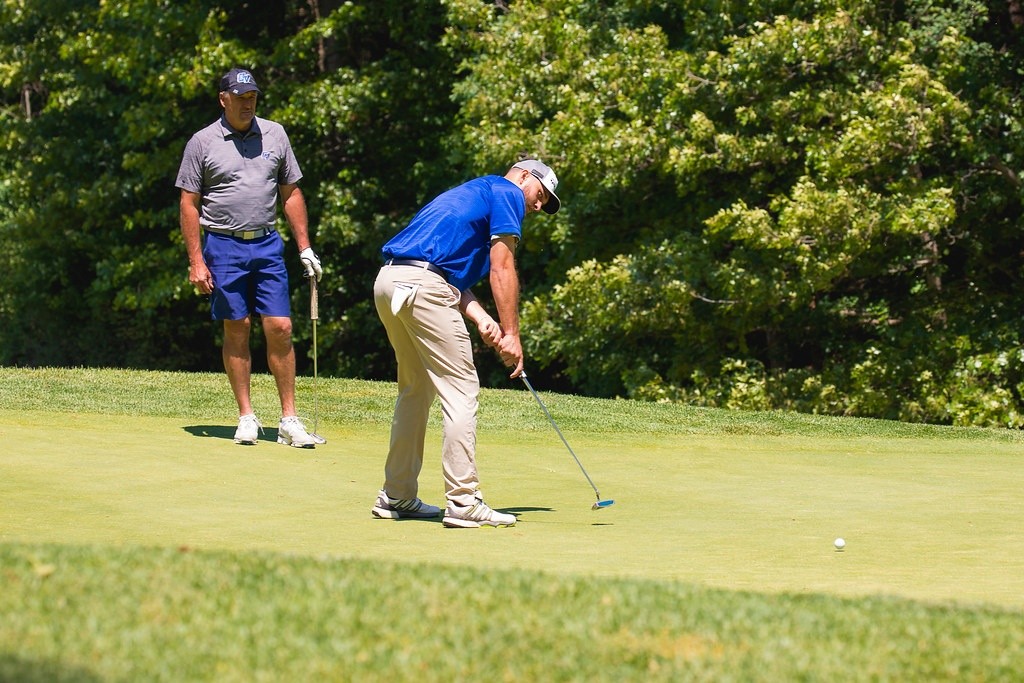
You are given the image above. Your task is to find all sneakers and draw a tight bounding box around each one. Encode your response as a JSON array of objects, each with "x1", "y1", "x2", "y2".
[
  {"x1": 372, "y1": 489, "x2": 441, "y2": 519},
  {"x1": 442, "y1": 497, "x2": 516, "y2": 528},
  {"x1": 276, "y1": 416, "x2": 315, "y2": 448},
  {"x1": 233, "y1": 414, "x2": 265, "y2": 445}
]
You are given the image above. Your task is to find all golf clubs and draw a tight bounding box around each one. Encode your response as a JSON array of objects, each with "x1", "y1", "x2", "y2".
[
  {"x1": 512, "y1": 361, "x2": 616, "y2": 512},
  {"x1": 311, "y1": 273, "x2": 327, "y2": 444}
]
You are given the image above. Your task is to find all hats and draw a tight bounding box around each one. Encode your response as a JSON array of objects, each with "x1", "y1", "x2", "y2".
[
  {"x1": 219, "y1": 68, "x2": 263, "y2": 97},
  {"x1": 511, "y1": 159, "x2": 562, "y2": 215}
]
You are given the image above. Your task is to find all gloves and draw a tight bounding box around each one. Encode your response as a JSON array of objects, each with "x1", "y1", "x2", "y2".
[{"x1": 298, "y1": 247, "x2": 323, "y2": 282}]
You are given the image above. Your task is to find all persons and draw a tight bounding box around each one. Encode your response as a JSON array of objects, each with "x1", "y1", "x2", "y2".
[
  {"x1": 368, "y1": 159, "x2": 560, "y2": 528},
  {"x1": 174, "y1": 68, "x2": 324, "y2": 448}
]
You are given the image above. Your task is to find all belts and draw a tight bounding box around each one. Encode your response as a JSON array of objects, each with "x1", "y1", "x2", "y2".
[
  {"x1": 384, "y1": 257, "x2": 448, "y2": 282},
  {"x1": 201, "y1": 224, "x2": 275, "y2": 239}
]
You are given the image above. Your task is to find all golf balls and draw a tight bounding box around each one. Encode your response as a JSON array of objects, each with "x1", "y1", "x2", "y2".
[{"x1": 834, "y1": 538, "x2": 846, "y2": 549}]
